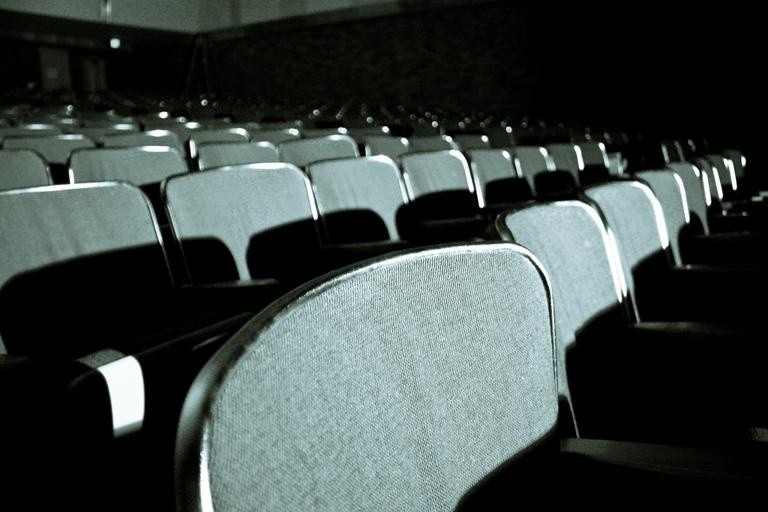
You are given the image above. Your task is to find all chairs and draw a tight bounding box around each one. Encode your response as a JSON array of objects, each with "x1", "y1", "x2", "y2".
[
  {"x1": 3, "y1": 181, "x2": 284, "y2": 445},
  {"x1": 583, "y1": 181, "x2": 766, "y2": 322},
  {"x1": 176, "y1": 242, "x2": 715, "y2": 510},
  {"x1": 2, "y1": 99, "x2": 768, "y2": 263},
  {"x1": 160, "y1": 161, "x2": 407, "y2": 293},
  {"x1": 495, "y1": 200, "x2": 766, "y2": 399}
]
